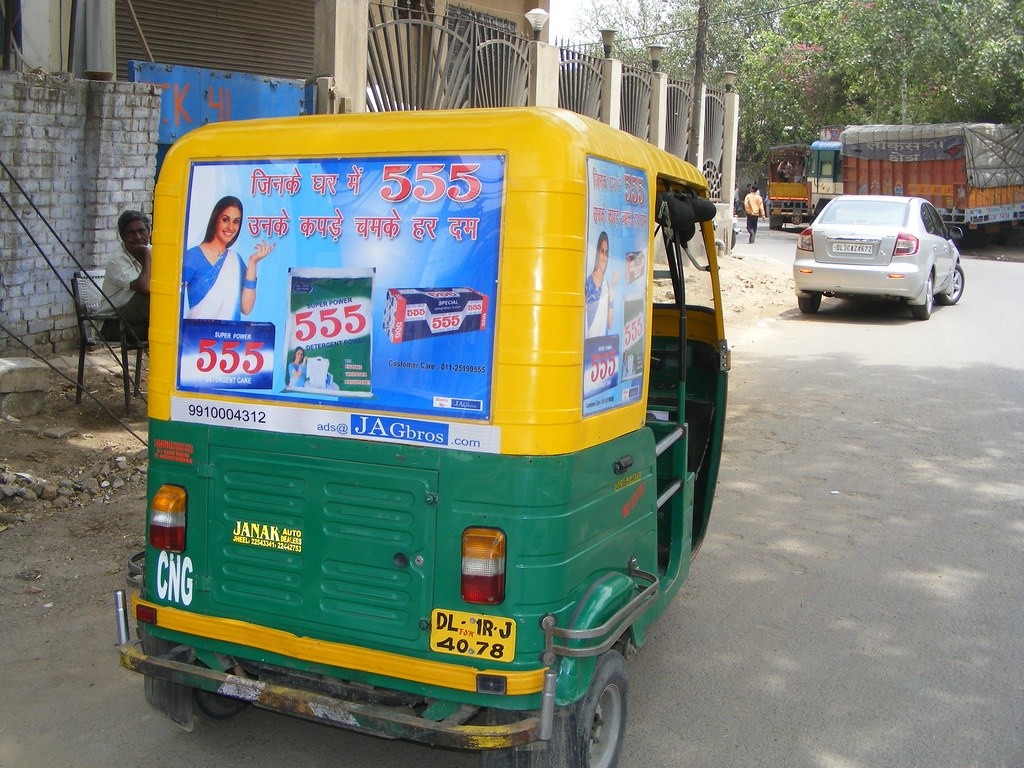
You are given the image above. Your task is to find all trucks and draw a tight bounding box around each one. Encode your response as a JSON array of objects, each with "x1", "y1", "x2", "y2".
[
  {"x1": 803, "y1": 120, "x2": 1024, "y2": 252},
  {"x1": 763, "y1": 143, "x2": 816, "y2": 231}
]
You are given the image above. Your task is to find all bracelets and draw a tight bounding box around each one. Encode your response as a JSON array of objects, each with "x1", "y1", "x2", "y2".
[
  {"x1": 608, "y1": 302, "x2": 614, "y2": 308},
  {"x1": 244, "y1": 278, "x2": 258, "y2": 289},
  {"x1": 300, "y1": 366, "x2": 305, "y2": 370}
]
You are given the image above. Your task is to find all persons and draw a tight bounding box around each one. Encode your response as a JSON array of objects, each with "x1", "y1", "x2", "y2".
[
  {"x1": 733, "y1": 184, "x2": 761, "y2": 215},
  {"x1": 777, "y1": 160, "x2": 806, "y2": 183},
  {"x1": 185, "y1": 196, "x2": 276, "y2": 321},
  {"x1": 744, "y1": 184, "x2": 765, "y2": 243},
  {"x1": 586, "y1": 231, "x2": 618, "y2": 338},
  {"x1": 96, "y1": 211, "x2": 151, "y2": 341},
  {"x1": 289, "y1": 346, "x2": 308, "y2": 387}
]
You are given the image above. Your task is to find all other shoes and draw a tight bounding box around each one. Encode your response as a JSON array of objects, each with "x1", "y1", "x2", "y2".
[{"x1": 749, "y1": 233, "x2": 755, "y2": 243}]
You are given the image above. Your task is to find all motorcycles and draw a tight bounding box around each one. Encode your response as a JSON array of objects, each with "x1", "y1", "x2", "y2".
[{"x1": 116, "y1": 100, "x2": 731, "y2": 768}]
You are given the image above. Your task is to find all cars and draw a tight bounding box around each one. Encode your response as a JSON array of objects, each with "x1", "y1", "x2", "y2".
[{"x1": 792, "y1": 194, "x2": 965, "y2": 322}]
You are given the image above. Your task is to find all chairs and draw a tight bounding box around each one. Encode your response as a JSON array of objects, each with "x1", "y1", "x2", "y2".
[{"x1": 69, "y1": 268, "x2": 150, "y2": 415}]
